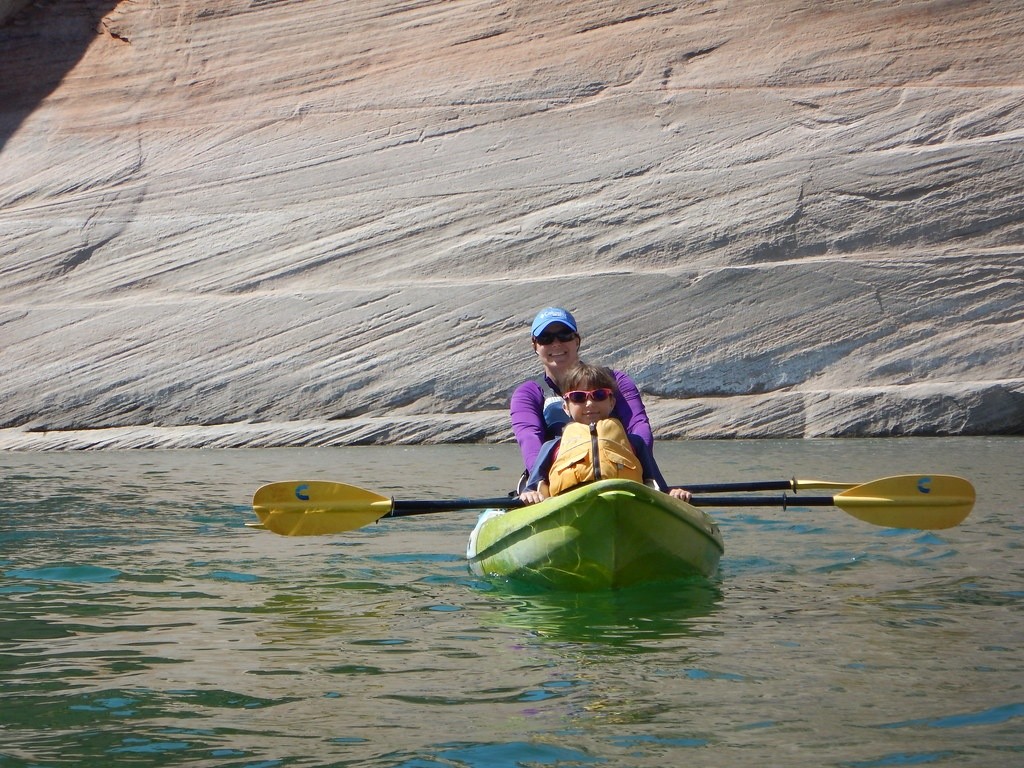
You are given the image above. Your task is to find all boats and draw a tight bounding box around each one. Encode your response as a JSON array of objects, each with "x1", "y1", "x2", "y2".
[{"x1": 465, "y1": 476, "x2": 727, "y2": 595}]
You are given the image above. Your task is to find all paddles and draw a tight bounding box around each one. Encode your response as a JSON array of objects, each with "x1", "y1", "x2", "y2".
[{"x1": 243, "y1": 472, "x2": 976, "y2": 536}]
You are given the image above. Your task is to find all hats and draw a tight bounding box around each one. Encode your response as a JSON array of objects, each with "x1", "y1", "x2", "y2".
[{"x1": 531, "y1": 306, "x2": 577, "y2": 337}]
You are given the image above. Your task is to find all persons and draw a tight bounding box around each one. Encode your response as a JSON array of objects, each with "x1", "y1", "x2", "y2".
[
  {"x1": 520, "y1": 364, "x2": 692, "y2": 509},
  {"x1": 510, "y1": 307, "x2": 661, "y2": 492}
]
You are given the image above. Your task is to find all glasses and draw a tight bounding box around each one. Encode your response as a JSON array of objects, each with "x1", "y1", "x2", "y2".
[
  {"x1": 562, "y1": 388, "x2": 615, "y2": 403},
  {"x1": 535, "y1": 328, "x2": 578, "y2": 346}
]
